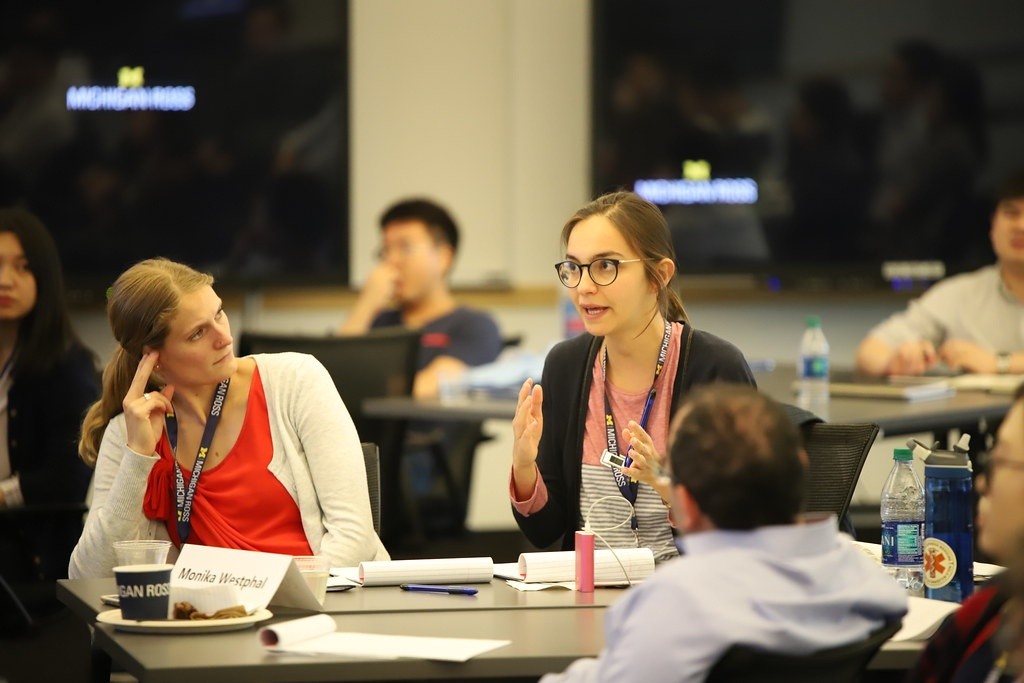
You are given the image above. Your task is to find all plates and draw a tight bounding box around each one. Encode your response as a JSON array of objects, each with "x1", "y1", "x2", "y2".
[{"x1": 96, "y1": 608, "x2": 273, "y2": 634}]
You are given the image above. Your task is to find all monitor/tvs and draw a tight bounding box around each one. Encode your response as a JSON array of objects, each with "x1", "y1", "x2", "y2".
[
  {"x1": 0, "y1": 0, "x2": 351, "y2": 293},
  {"x1": 590, "y1": 0, "x2": 1024, "y2": 291}
]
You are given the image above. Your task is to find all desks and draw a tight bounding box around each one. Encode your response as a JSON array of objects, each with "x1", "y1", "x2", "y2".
[
  {"x1": 361, "y1": 363, "x2": 1016, "y2": 564},
  {"x1": 57, "y1": 543, "x2": 984, "y2": 682}
]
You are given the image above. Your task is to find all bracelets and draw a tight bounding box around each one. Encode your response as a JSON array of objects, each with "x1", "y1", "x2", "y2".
[{"x1": 999, "y1": 351, "x2": 1010, "y2": 374}]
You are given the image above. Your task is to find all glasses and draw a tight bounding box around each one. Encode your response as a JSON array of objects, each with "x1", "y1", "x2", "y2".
[
  {"x1": 978, "y1": 451, "x2": 1024, "y2": 474},
  {"x1": 654, "y1": 454, "x2": 671, "y2": 479},
  {"x1": 555, "y1": 258, "x2": 658, "y2": 288}
]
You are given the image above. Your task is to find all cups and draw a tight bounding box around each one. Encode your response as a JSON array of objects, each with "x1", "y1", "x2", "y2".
[
  {"x1": 112, "y1": 561, "x2": 174, "y2": 620},
  {"x1": 112, "y1": 539, "x2": 172, "y2": 565}
]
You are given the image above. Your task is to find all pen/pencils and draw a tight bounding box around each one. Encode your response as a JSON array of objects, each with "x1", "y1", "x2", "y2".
[{"x1": 400, "y1": 583, "x2": 479, "y2": 595}]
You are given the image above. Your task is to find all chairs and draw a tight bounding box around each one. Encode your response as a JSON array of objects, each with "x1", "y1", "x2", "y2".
[
  {"x1": 794, "y1": 421, "x2": 882, "y2": 542},
  {"x1": 706, "y1": 622, "x2": 901, "y2": 683},
  {"x1": 237, "y1": 331, "x2": 423, "y2": 557}
]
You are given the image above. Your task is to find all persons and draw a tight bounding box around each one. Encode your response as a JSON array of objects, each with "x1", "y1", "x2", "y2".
[
  {"x1": 539, "y1": 380, "x2": 908, "y2": 683},
  {"x1": 340, "y1": 200, "x2": 502, "y2": 498},
  {"x1": 67, "y1": 256, "x2": 393, "y2": 649},
  {"x1": 855, "y1": 184, "x2": 1024, "y2": 375},
  {"x1": 508, "y1": 190, "x2": 756, "y2": 554},
  {"x1": 917, "y1": 382, "x2": 1024, "y2": 683},
  {"x1": 0, "y1": 200, "x2": 103, "y2": 638}
]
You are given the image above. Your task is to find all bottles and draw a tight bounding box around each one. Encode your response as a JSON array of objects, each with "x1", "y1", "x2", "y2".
[
  {"x1": 907, "y1": 434, "x2": 974, "y2": 602},
  {"x1": 881, "y1": 448, "x2": 925, "y2": 598},
  {"x1": 798, "y1": 316, "x2": 831, "y2": 426}
]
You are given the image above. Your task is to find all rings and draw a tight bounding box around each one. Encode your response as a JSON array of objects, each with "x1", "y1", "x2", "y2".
[{"x1": 144, "y1": 393, "x2": 151, "y2": 400}]
